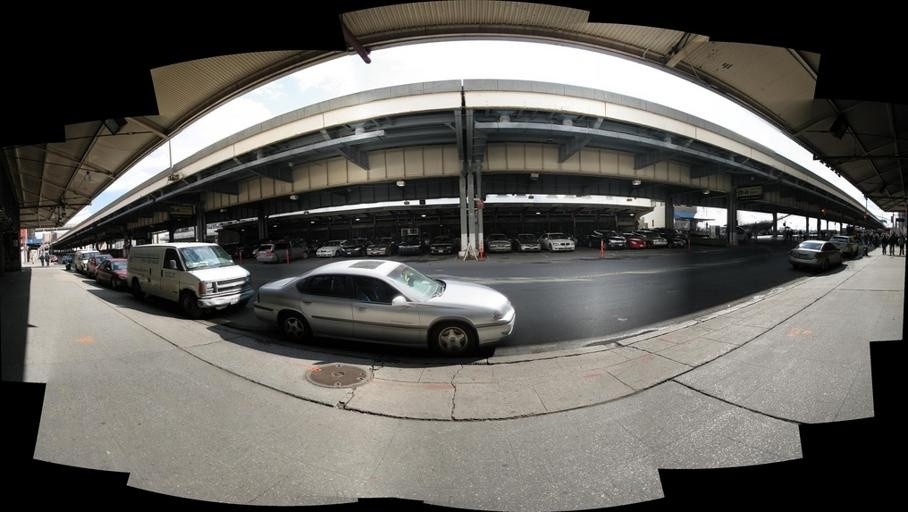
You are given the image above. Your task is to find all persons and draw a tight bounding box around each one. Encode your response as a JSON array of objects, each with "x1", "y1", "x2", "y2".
[
  {"x1": 39, "y1": 255, "x2": 45, "y2": 266},
  {"x1": 881, "y1": 232, "x2": 905, "y2": 256},
  {"x1": 45, "y1": 253, "x2": 50, "y2": 266}
]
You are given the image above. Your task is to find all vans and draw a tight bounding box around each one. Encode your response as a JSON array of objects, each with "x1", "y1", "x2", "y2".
[
  {"x1": 74, "y1": 249, "x2": 100, "y2": 274},
  {"x1": 256, "y1": 242, "x2": 309, "y2": 264}
]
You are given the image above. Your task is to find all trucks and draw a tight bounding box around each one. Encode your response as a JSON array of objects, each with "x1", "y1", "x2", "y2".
[{"x1": 126, "y1": 240, "x2": 255, "y2": 316}]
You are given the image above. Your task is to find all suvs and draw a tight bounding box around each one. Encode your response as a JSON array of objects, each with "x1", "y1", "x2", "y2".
[{"x1": 829, "y1": 234, "x2": 867, "y2": 258}]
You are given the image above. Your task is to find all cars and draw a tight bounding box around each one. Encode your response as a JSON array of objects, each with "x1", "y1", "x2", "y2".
[
  {"x1": 46, "y1": 255, "x2": 58, "y2": 262},
  {"x1": 253, "y1": 258, "x2": 517, "y2": 356},
  {"x1": 86, "y1": 254, "x2": 114, "y2": 279},
  {"x1": 61, "y1": 256, "x2": 72, "y2": 264},
  {"x1": 719, "y1": 225, "x2": 751, "y2": 242},
  {"x1": 94, "y1": 258, "x2": 128, "y2": 289},
  {"x1": 788, "y1": 239, "x2": 844, "y2": 272},
  {"x1": 486, "y1": 227, "x2": 691, "y2": 254},
  {"x1": 315, "y1": 232, "x2": 455, "y2": 257}
]
